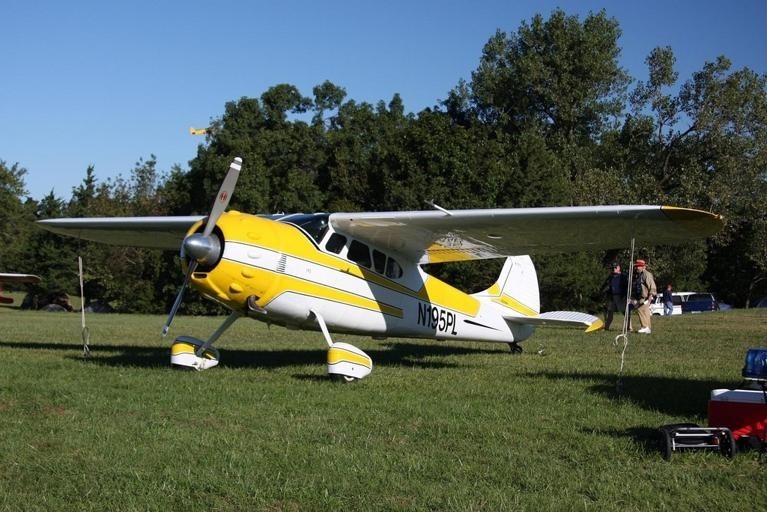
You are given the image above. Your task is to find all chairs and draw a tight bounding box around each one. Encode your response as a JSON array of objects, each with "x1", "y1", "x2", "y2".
[{"x1": 738, "y1": 347, "x2": 767, "y2": 391}]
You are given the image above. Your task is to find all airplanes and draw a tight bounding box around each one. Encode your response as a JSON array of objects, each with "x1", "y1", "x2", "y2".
[
  {"x1": 0, "y1": 272, "x2": 42, "y2": 308},
  {"x1": 33, "y1": 156, "x2": 729, "y2": 385}
]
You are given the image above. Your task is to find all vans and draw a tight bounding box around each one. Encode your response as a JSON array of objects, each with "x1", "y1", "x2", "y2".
[{"x1": 649, "y1": 291, "x2": 697, "y2": 316}]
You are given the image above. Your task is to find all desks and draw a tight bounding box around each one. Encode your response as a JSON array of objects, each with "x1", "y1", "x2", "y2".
[{"x1": 708, "y1": 389, "x2": 767, "y2": 452}]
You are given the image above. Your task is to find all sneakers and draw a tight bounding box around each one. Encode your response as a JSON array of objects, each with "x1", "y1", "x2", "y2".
[{"x1": 637, "y1": 326, "x2": 652, "y2": 335}]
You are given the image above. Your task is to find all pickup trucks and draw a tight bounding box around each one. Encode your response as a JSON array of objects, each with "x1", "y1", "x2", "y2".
[{"x1": 681, "y1": 291, "x2": 720, "y2": 314}]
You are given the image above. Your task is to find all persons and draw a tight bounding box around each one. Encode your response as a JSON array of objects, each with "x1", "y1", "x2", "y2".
[
  {"x1": 600, "y1": 262, "x2": 637, "y2": 331},
  {"x1": 663, "y1": 284, "x2": 674, "y2": 316},
  {"x1": 633, "y1": 259, "x2": 657, "y2": 334}
]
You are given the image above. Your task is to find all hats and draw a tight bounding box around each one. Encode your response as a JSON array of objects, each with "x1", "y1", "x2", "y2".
[
  {"x1": 612, "y1": 263, "x2": 619, "y2": 267},
  {"x1": 633, "y1": 259, "x2": 646, "y2": 266}
]
